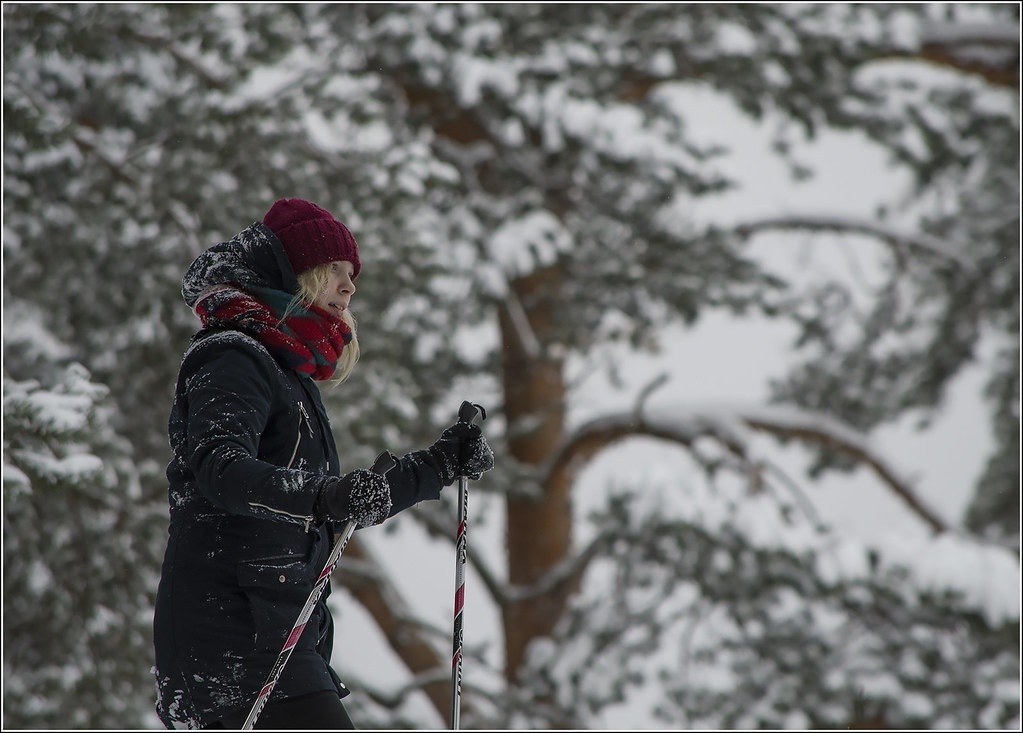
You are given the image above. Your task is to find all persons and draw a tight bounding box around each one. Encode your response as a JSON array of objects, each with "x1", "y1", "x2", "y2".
[{"x1": 151, "y1": 197, "x2": 495, "y2": 730}]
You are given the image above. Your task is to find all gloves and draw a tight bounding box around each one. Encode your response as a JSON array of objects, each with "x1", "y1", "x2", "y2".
[
  {"x1": 323, "y1": 469, "x2": 391, "y2": 528},
  {"x1": 429, "y1": 421, "x2": 496, "y2": 487}
]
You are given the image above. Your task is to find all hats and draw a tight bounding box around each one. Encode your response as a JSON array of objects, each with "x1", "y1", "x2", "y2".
[{"x1": 264, "y1": 198, "x2": 361, "y2": 283}]
